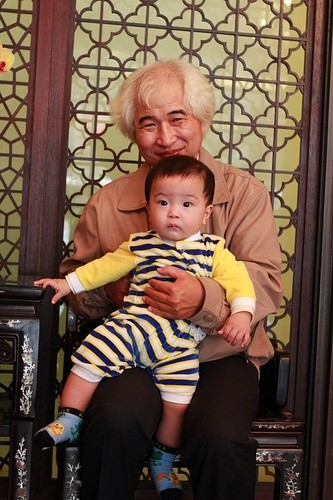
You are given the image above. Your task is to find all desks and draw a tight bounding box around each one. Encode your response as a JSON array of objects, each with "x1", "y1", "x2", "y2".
[{"x1": 0, "y1": 272, "x2": 62, "y2": 500}]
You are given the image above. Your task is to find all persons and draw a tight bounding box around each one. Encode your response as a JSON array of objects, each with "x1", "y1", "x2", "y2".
[
  {"x1": 54, "y1": 57, "x2": 285, "y2": 500},
  {"x1": 31, "y1": 153, "x2": 257, "y2": 500}
]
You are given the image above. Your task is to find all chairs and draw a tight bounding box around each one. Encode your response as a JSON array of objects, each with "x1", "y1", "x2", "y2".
[{"x1": 60, "y1": 296, "x2": 305, "y2": 500}]
